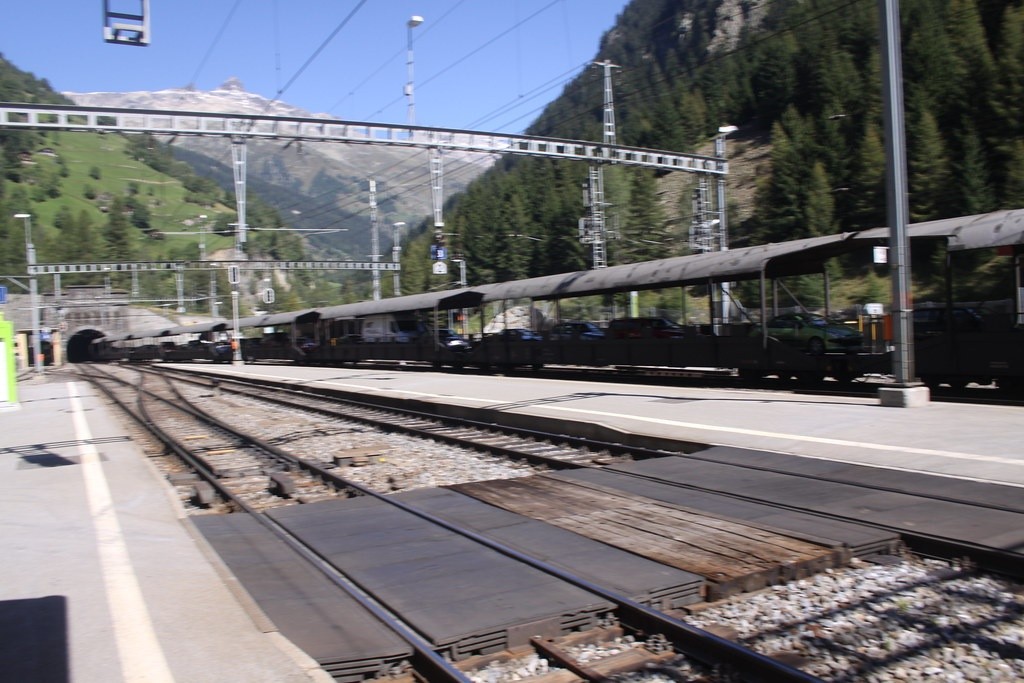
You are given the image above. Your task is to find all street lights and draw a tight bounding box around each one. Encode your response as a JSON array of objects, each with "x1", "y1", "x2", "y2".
[
  {"x1": 13, "y1": 213, "x2": 44, "y2": 375},
  {"x1": 404, "y1": 11, "x2": 426, "y2": 123}
]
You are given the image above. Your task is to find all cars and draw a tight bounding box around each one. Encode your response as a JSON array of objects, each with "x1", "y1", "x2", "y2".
[{"x1": 117, "y1": 316, "x2": 879, "y2": 363}]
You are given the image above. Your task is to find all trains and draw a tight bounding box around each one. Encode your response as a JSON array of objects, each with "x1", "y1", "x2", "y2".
[{"x1": 87, "y1": 206, "x2": 1024, "y2": 401}]
